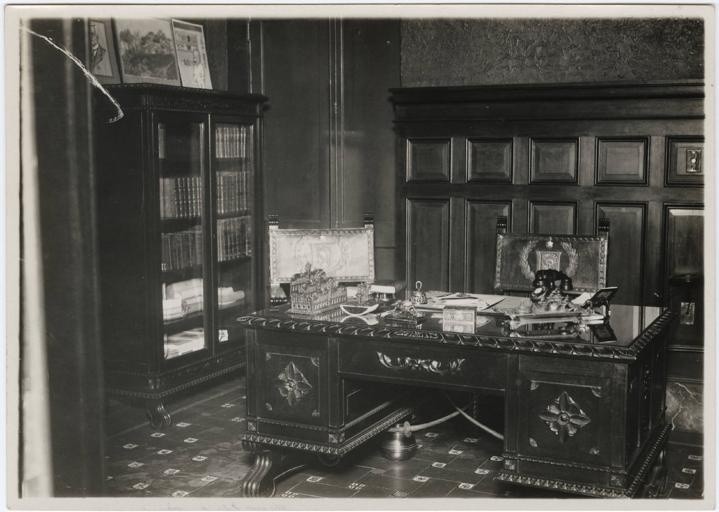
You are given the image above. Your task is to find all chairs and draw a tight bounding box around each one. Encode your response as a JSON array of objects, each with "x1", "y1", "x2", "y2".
[{"x1": 493, "y1": 214, "x2": 611, "y2": 300}]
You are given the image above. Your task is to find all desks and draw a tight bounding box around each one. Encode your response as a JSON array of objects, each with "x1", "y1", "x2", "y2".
[{"x1": 236, "y1": 290, "x2": 675, "y2": 497}]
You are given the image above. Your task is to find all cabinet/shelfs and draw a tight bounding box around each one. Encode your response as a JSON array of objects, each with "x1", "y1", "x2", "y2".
[
  {"x1": 386, "y1": 77, "x2": 704, "y2": 386},
  {"x1": 502, "y1": 322, "x2": 676, "y2": 497},
  {"x1": 97, "y1": 85, "x2": 273, "y2": 402},
  {"x1": 238, "y1": 322, "x2": 440, "y2": 456}
]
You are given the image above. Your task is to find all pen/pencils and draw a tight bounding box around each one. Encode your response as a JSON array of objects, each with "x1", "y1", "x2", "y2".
[{"x1": 442, "y1": 297, "x2": 478, "y2": 300}]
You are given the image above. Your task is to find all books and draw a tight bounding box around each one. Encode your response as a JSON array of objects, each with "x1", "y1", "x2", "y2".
[{"x1": 158, "y1": 121, "x2": 251, "y2": 358}]
